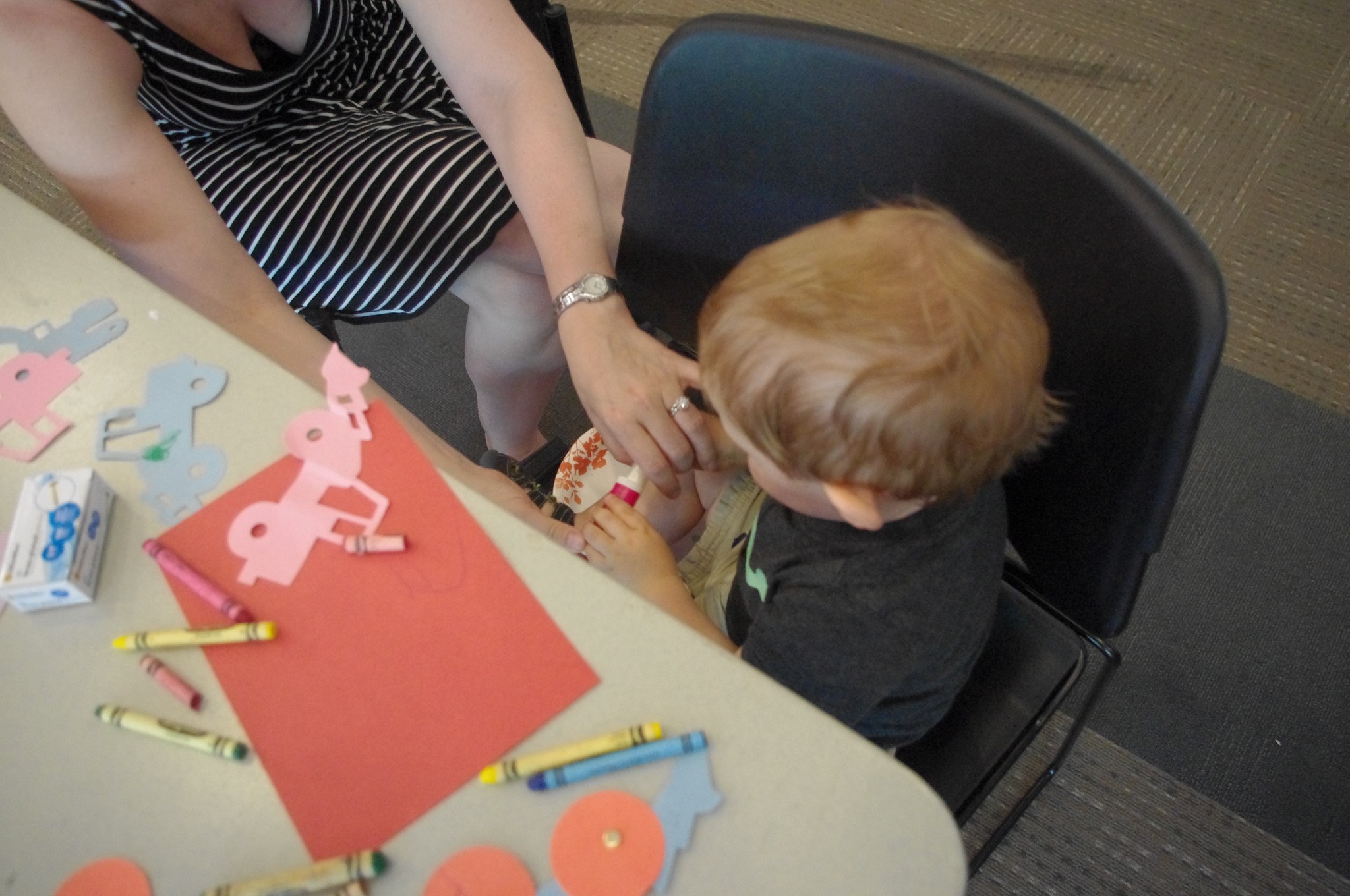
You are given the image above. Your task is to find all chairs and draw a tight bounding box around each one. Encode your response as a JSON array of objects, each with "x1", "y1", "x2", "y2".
[{"x1": 526, "y1": 11, "x2": 1228, "y2": 867}]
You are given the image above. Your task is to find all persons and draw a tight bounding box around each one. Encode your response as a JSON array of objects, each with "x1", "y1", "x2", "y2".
[
  {"x1": 1, "y1": 1, "x2": 722, "y2": 561},
  {"x1": 477, "y1": 196, "x2": 1073, "y2": 748}
]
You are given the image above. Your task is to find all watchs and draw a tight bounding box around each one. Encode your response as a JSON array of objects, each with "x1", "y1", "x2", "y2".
[{"x1": 552, "y1": 273, "x2": 626, "y2": 319}]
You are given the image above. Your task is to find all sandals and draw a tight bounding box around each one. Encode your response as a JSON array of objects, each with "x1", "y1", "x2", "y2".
[
  {"x1": 518, "y1": 436, "x2": 570, "y2": 496},
  {"x1": 479, "y1": 449, "x2": 576, "y2": 527}
]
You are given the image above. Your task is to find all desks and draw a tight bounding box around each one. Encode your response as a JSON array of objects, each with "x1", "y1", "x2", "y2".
[{"x1": 0, "y1": 181, "x2": 971, "y2": 896}]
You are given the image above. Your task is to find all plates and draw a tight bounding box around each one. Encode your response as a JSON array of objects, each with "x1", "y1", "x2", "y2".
[{"x1": 552, "y1": 422, "x2": 650, "y2": 565}]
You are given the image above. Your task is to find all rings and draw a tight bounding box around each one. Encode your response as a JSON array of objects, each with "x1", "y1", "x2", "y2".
[{"x1": 669, "y1": 395, "x2": 690, "y2": 417}]
